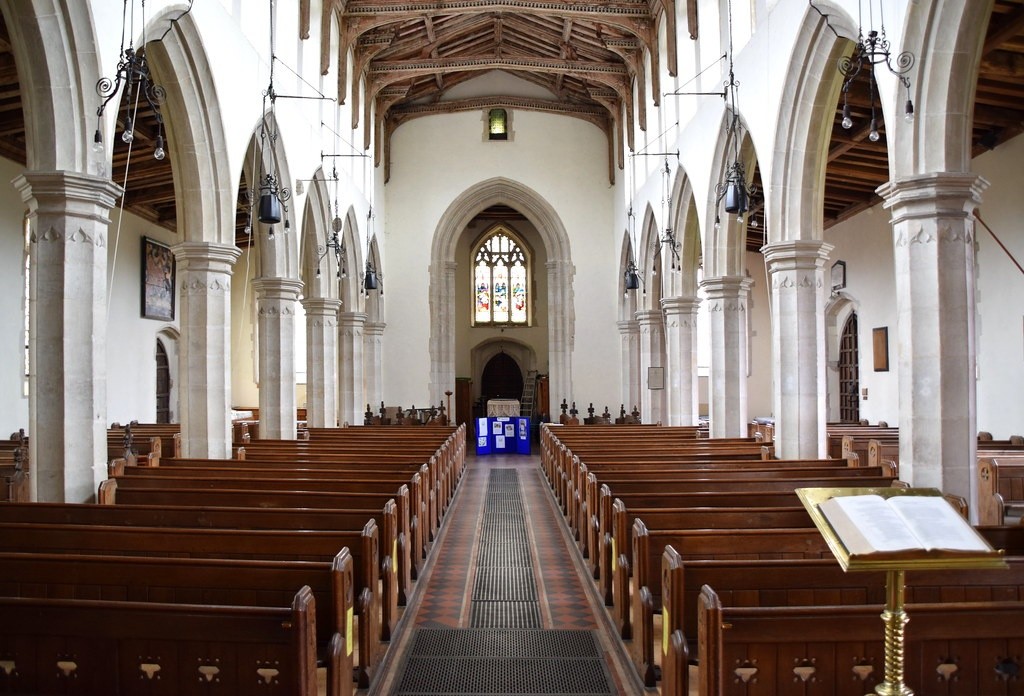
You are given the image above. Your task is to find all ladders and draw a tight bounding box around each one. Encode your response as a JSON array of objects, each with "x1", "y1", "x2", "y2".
[{"x1": 520, "y1": 370, "x2": 538, "y2": 424}]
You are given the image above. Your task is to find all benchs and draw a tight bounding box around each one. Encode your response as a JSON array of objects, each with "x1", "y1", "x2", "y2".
[
  {"x1": 539, "y1": 421, "x2": 1023, "y2": 696},
  {"x1": 0, "y1": 422, "x2": 466, "y2": 696}
]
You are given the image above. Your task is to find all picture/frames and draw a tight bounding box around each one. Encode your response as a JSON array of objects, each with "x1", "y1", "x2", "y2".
[{"x1": 142, "y1": 235, "x2": 176, "y2": 322}]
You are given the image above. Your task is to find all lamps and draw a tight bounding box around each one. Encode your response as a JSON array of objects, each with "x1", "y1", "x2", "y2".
[
  {"x1": 94, "y1": 0, "x2": 167, "y2": 160},
  {"x1": 245, "y1": 0, "x2": 291, "y2": 240},
  {"x1": 624, "y1": 152, "x2": 680, "y2": 299},
  {"x1": 321, "y1": 154, "x2": 384, "y2": 299},
  {"x1": 837, "y1": 0, "x2": 914, "y2": 142},
  {"x1": 296, "y1": 178, "x2": 346, "y2": 281},
  {"x1": 651, "y1": 89, "x2": 728, "y2": 275},
  {"x1": 715, "y1": 0, "x2": 758, "y2": 229}
]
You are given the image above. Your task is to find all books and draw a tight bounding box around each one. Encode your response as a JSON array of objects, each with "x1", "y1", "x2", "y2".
[{"x1": 817, "y1": 495, "x2": 995, "y2": 558}]
